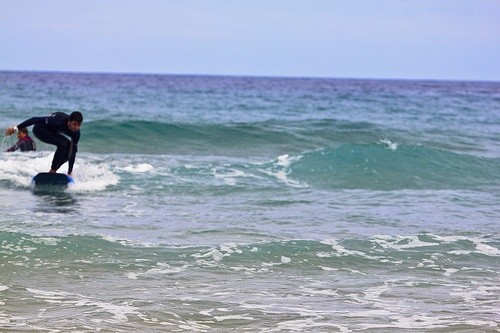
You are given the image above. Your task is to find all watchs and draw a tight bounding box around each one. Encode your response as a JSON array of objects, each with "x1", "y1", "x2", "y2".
[{"x1": 14, "y1": 125, "x2": 19, "y2": 133}]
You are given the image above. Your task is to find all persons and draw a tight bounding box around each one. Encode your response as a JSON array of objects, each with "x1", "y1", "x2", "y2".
[
  {"x1": 3, "y1": 126, "x2": 37, "y2": 153},
  {"x1": 4, "y1": 110, "x2": 84, "y2": 177}
]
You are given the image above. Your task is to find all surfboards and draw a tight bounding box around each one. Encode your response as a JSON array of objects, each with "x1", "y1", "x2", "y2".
[{"x1": 32, "y1": 173, "x2": 75, "y2": 187}]
